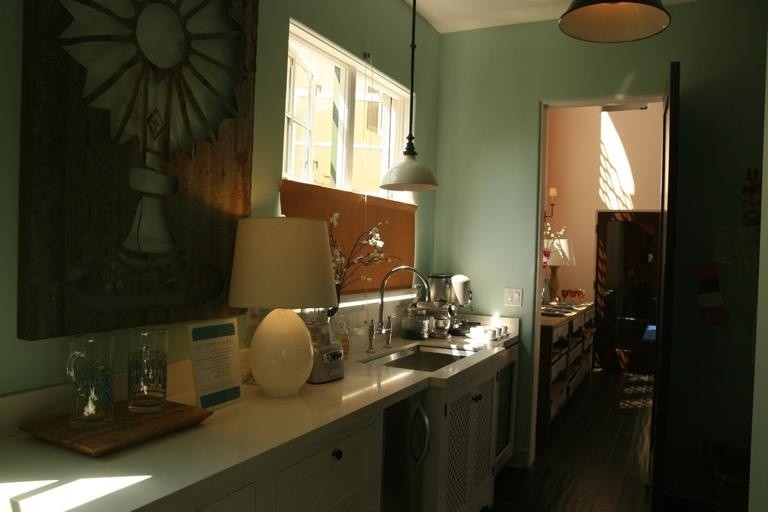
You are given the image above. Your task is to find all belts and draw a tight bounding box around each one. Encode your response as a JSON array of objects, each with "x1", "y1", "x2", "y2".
[{"x1": 561, "y1": 288, "x2": 584, "y2": 303}]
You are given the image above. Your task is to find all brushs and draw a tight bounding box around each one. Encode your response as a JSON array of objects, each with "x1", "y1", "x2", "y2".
[{"x1": 427, "y1": 272, "x2": 481, "y2": 335}]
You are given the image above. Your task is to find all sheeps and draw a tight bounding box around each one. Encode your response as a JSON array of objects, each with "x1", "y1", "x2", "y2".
[{"x1": 375, "y1": 265, "x2": 432, "y2": 333}]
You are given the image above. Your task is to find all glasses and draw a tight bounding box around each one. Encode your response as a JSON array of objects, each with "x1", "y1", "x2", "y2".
[
  {"x1": 542, "y1": 239, "x2": 575, "y2": 303},
  {"x1": 230, "y1": 213, "x2": 340, "y2": 397},
  {"x1": 544, "y1": 186, "x2": 558, "y2": 218},
  {"x1": 381, "y1": 2, "x2": 438, "y2": 192},
  {"x1": 558, "y1": 0, "x2": 670, "y2": 42}
]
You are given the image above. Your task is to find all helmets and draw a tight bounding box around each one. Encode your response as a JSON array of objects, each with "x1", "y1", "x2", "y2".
[{"x1": 542, "y1": 304, "x2": 573, "y2": 316}]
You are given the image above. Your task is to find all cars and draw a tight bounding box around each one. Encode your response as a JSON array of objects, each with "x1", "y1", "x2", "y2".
[
  {"x1": 126, "y1": 327, "x2": 169, "y2": 412},
  {"x1": 67, "y1": 334, "x2": 115, "y2": 429}
]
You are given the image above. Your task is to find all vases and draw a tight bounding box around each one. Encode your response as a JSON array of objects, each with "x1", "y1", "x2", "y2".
[{"x1": 328, "y1": 211, "x2": 399, "y2": 291}]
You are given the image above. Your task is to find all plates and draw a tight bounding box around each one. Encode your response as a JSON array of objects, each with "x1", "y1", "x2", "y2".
[
  {"x1": 424, "y1": 362, "x2": 502, "y2": 512},
  {"x1": 542, "y1": 305, "x2": 594, "y2": 428},
  {"x1": 266, "y1": 408, "x2": 384, "y2": 510}
]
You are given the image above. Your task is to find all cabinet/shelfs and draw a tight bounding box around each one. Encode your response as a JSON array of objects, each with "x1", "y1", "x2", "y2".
[
  {"x1": 309, "y1": 315, "x2": 349, "y2": 385},
  {"x1": 541, "y1": 279, "x2": 550, "y2": 305},
  {"x1": 402, "y1": 308, "x2": 450, "y2": 340}
]
